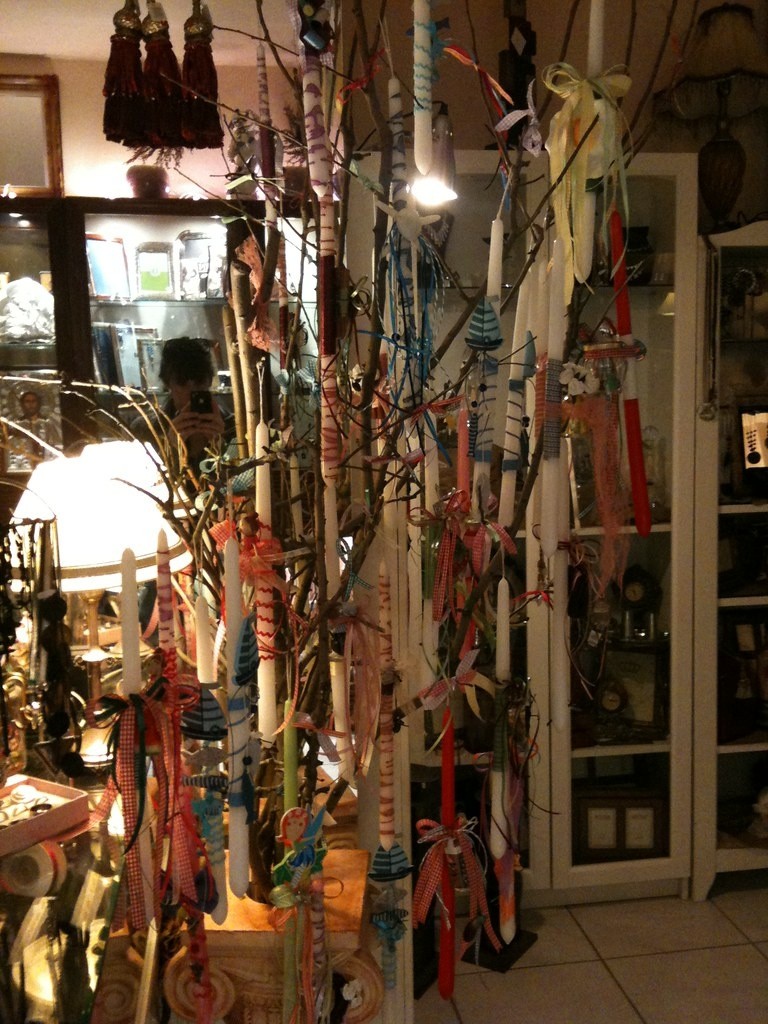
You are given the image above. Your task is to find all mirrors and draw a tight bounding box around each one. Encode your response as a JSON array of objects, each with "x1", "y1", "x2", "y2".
[{"x1": 0, "y1": 73, "x2": 66, "y2": 198}]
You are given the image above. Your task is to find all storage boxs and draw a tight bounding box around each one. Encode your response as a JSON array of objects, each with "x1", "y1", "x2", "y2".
[{"x1": 0, "y1": 778, "x2": 89, "y2": 857}]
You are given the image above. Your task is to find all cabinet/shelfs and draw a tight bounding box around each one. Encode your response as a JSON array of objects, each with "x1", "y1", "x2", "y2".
[
  {"x1": 694, "y1": 219, "x2": 768, "y2": 903},
  {"x1": 410, "y1": 147, "x2": 699, "y2": 921},
  {"x1": 0, "y1": 198, "x2": 342, "y2": 452}
]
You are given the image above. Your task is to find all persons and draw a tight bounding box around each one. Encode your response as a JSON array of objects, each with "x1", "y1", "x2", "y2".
[
  {"x1": 13, "y1": 393, "x2": 60, "y2": 452},
  {"x1": 128, "y1": 336, "x2": 237, "y2": 648}
]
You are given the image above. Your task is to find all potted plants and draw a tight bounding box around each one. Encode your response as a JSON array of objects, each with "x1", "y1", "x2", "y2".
[
  {"x1": 125, "y1": 146, "x2": 184, "y2": 198},
  {"x1": 278, "y1": 67, "x2": 310, "y2": 199},
  {"x1": 226, "y1": 110, "x2": 262, "y2": 200}
]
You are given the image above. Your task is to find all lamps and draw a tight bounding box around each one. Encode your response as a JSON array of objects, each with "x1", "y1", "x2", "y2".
[
  {"x1": 7, "y1": 439, "x2": 198, "y2": 662},
  {"x1": 406, "y1": 104, "x2": 460, "y2": 205}
]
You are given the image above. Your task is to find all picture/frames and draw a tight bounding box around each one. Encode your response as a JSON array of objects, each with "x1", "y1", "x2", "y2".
[
  {"x1": 93, "y1": 323, "x2": 125, "y2": 388},
  {"x1": 113, "y1": 324, "x2": 159, "y2": 388},
  {"x1": 137, "y1": 338, "x2": 227, "y2": 390}
]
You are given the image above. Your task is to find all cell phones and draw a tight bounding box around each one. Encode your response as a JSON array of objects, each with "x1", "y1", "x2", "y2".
[{"x1": 191, "y1": 391, "x2": 214, "y2": 428}]
